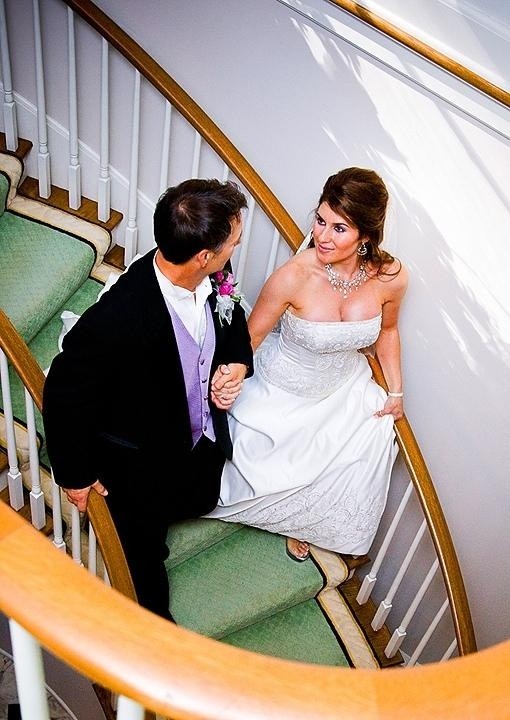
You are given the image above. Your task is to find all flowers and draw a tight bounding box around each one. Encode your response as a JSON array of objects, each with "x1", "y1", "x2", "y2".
[{"x1": 210, "y1": 268, "x2": 241, "y2": 327}]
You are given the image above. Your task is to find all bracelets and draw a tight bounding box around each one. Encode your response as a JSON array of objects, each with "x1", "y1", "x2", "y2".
[{"x1": 386, "y1": 390, "x2": 402, "y2": 398}]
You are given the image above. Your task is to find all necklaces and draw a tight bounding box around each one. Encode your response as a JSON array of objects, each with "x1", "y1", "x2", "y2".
[{"x1": 324, "y1": 261, "x2": 370, "y2": 298}]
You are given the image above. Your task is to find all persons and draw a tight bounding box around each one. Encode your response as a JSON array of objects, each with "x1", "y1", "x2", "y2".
[
  {"x1": 42, "y1": 175, "x2": 255, "y2": 652},
  {"x1": 210, "y1": 165, "x2": 408, "y2": 566}
]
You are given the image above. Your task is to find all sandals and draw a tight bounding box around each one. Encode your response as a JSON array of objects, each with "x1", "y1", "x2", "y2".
[{"x1": 285, "y1": 537, "x2": 310, "y2": 563}]
